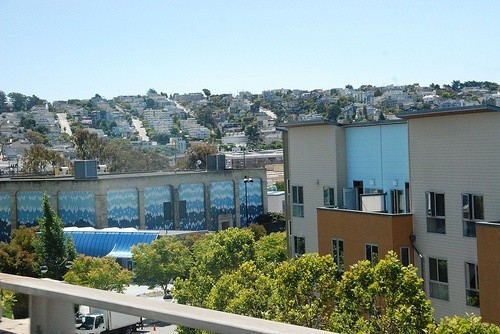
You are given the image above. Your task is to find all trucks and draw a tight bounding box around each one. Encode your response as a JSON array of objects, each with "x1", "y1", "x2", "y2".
[{"x1": 76, "y1": 305, "x2": 141, "y2": 334}]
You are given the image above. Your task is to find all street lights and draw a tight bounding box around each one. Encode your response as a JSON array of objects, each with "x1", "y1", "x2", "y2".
[{"x1": 243, "y1": 175, "x2": 253, "y2": 227}]
